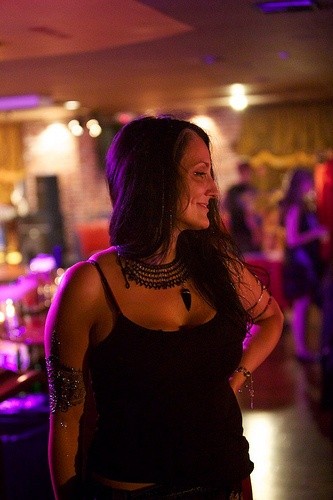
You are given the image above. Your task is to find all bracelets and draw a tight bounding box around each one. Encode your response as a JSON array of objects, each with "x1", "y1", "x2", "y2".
[{"x1": 232, "y1": 366, "x2": 255, "y2": 409}]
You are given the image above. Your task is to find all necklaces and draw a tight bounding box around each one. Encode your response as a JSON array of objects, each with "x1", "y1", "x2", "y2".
[{"x1": 112, "y1": 243, "x2": 194, "y2": 315}]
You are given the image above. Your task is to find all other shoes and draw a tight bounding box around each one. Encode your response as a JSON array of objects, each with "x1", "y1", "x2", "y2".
[{"x1": 290, "y1": 352, "x2": 315, "y2": 362}]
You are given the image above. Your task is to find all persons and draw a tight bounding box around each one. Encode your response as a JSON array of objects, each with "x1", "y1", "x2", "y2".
[
  {"x1": 220, "y1": 161, "x2": 265, "y2": 254},
  {"x1": 42, "y1": 115, "x2": 283, "y2": 500},
  {"x1": 277, "y1": 168, "x2": 331, "y2": 365}
]
